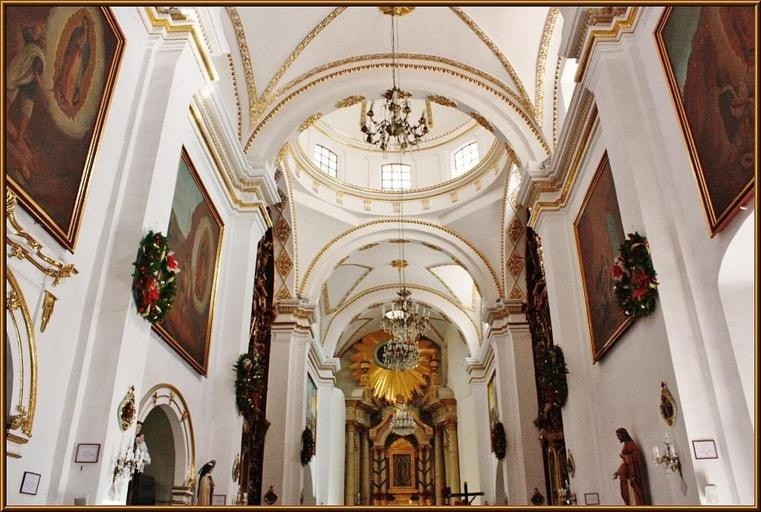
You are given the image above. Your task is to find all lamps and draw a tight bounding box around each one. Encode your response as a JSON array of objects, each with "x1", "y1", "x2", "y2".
[
  {"x1": 115, "y1": 434, "x2": 152, "y2": 478},
  {"x1": 360, "y1": 6, "x2": 431, "y2": 436},
  {"x1": 647, "y1": 429, "x2": 683, "y2": 480}
]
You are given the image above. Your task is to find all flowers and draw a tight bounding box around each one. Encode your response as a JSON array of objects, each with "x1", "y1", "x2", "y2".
[
  {"x1": 492, "y1": 422, "x2": 507, "y2": 461},
  {"x1": 612, "y1": 231, "x2": 659, "y2": 321},
  {"x1": 131, "y1": 231, "x2": 182, "y2": 325},
  {"x1": 233, "y1": 353, "x2": 266, "y2": 417},
  {"x1": 539, "y1": 344, "x2": 570, "y2": 409},
  {"x1": 301, "y1": 426, "x2": 316, "y2": 468}
]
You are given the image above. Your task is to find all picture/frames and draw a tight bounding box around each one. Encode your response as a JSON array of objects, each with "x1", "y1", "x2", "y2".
[
  {"x1": 75, "y1": 443, "x2": 101, "y2": 463},
  {"x1": 152, "y1": 143, "x2": 225, "y2": 380},
  {"x1": 573, "y1": 147, "x2": 637, "y2": 365},
  {"x1": 19, "y1": 471, "x2": 41, "y2": 495},
  {"x1": 653, "y1": 6, "x2": 755, "y2": 238},
  {"x1": 487, "y1": 368, "x2": 499, "y2": 453},
  {"x1": 212, "y1": 495, "x2": 226, "y2": 506},
  {"x1": 692, "y1": 439, "x2": 718, "y2": 459},
  {"x1": 5, "y1": 4, "x2": 127, "y2": 254},
  {"x1": 306, "y1": 371, "x2": 317, "y2": 456},
  {"x1": 585, "y1": 493, "x2": 599, "y2": 505}
]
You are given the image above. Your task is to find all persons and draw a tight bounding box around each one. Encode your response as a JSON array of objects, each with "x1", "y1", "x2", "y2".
[
  {"x1": 196, "y1": 459, "x2": 218, "y2": 505},
  {"x1": 59, "y1": 15, "x2": 91, "y2": 106},
  {"x1": 612, "y1": 426, "x2": 652, "y2": 505},
  {"x1": 8, "y1": 20, "x2": 50, "y2": 152},
  {"x1": 715, "y1": 69, "x2": 756, "y2": 171}
]
